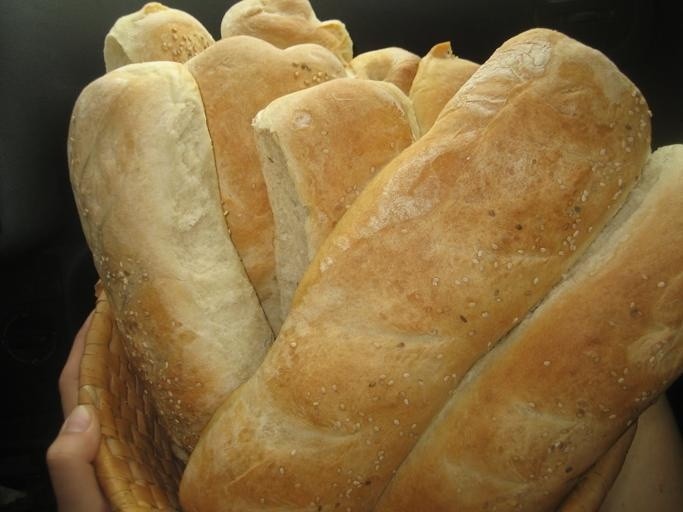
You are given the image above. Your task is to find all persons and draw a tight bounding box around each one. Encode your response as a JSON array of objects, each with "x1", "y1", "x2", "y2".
[{"x1": 47, "y1": 308, "x2": 107, "y2": 509}]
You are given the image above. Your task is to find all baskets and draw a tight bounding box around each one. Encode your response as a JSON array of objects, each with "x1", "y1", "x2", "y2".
[{"x1": 78, "y1": 289, "x2": 638, "y2": 512}]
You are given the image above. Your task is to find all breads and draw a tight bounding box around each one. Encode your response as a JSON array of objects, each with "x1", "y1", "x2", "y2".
[{"x1": 67, "y1": 0, "x2": 683, "y2": 512}]
